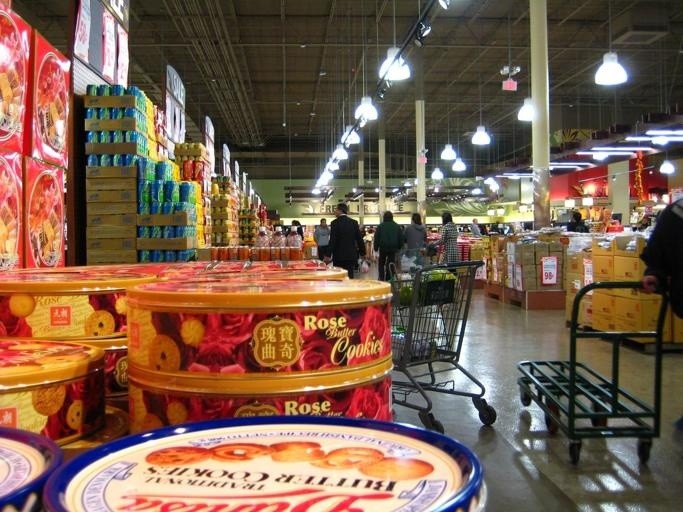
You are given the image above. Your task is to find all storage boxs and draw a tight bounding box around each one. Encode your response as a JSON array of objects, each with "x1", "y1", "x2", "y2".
[{"x1": 408, "y1": 231, "x2": 683, "y2": 354}]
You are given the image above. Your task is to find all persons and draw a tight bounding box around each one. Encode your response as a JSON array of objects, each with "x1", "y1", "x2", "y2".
[
  {"x1": 372, "y1": 210, "x2": 404, "y2": 283},
  {"x1": 639, "y1": 196, "x2": 683, "y2": 321},
  {"x1": 321, "y1": 201, "x2": 366, "y2": 281},
  {"x1": 566, "y1": 212, "x2": 589, "y2": 232},
  {"x1": 470, "y1": 217, "x2": 481, "y2": 235},
  {"x1": 290, "y1": 218, "x2": 303, "y2": 239},
  {"x1": 402, "y1": 212, "x2": 425, "y2": 253},
  {"x1": 312, "y1": 218, "x2": 329, "y2": 261},
  {"x1": 428, "y1": 211, "x2": 459, "y2": 265}
]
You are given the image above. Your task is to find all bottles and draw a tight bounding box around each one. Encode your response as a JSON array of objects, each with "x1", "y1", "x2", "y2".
[{"x1": 256, "y1": 226, "x2": 319, "y2": 260}]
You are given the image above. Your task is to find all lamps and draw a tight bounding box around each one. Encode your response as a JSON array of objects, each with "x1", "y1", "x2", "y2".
[
  {"x1": 350, "y1": 1, "x2": 378, "y2": 121},
  {"x1": 379, "y1": 0, "x2": 414, "y2": 83},
  {"x1": 593, "y1": 1, "x2": 630, "y2": 90},
  {"x1": 429, "y1": 10, "x2": 536, "y2": 183},
  {"x1": 374, "y1": 0, "x2": 453, "y2": 102},
  {"x1": 659, "y1": 150, "x2": 675, "y2": 175},
  {"x1": 302, "y1": 1, "x2": 362, "y2": 197}
]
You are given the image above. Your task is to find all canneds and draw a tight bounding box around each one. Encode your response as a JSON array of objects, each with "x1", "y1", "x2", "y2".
[{"x1": 86, "y1": 86, "x2": 302, "y2": 261}]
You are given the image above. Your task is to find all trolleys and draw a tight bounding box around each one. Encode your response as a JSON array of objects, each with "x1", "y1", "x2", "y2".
[
  {"x1": 516, "y1": 281, "x2": 669, "y2": 466},
  {"x1": 386, "y1": 260, "x2": 496, "y2": 436},
  {"x1": 394, "y1": 240, "x2": 442, "y2": 273}
]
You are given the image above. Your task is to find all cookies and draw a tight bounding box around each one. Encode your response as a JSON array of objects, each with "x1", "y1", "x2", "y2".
[{"x1": 8, "y1": 294, "x2": 433, "y2": 482}]
